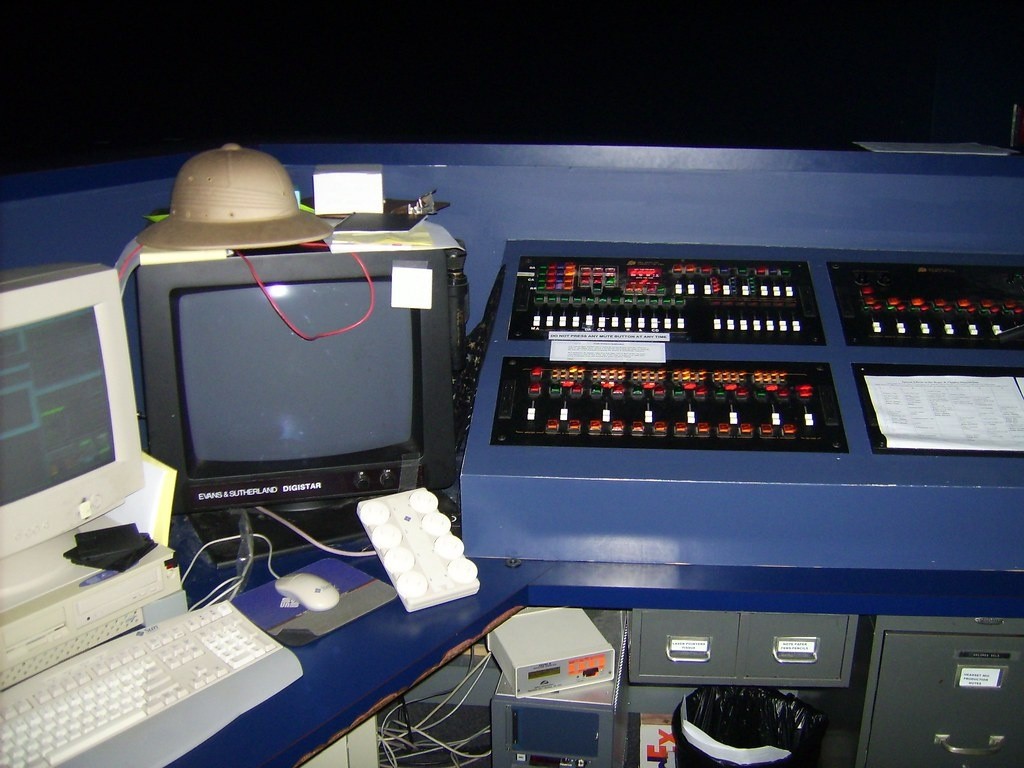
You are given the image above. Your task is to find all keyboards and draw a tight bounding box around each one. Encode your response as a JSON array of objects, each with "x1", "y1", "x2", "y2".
[{"x1": 0, "y1": 600, "x2": 302, "y2": 768}]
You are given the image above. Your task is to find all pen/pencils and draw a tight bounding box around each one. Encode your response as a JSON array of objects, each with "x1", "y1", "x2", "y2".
[{"x1": 78, "y1": 560, "x2": 140, "y2": 587}]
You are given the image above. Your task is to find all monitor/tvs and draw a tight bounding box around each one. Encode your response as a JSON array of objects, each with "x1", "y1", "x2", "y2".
[
  {"x1": 0, "y1": 261, "x2": 146, "y2": 615},
  {"x1": 133, "y1": 223, "x2": 457, "y2": 517}
]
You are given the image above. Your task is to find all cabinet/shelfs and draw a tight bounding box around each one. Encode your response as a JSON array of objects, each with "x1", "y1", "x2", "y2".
[{"x1": 855, "y1": 615, "x2": 1024, "y2": 768}]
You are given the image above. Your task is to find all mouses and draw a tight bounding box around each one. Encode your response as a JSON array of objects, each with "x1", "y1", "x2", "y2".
[{"x1": 275, "y1": 573, "x2": 340, "y2": 612}]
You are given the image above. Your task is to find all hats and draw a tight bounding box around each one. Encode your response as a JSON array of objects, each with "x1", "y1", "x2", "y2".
[{"x1": 134, "y1": 143, "x2": 335, "y2": 251}]
